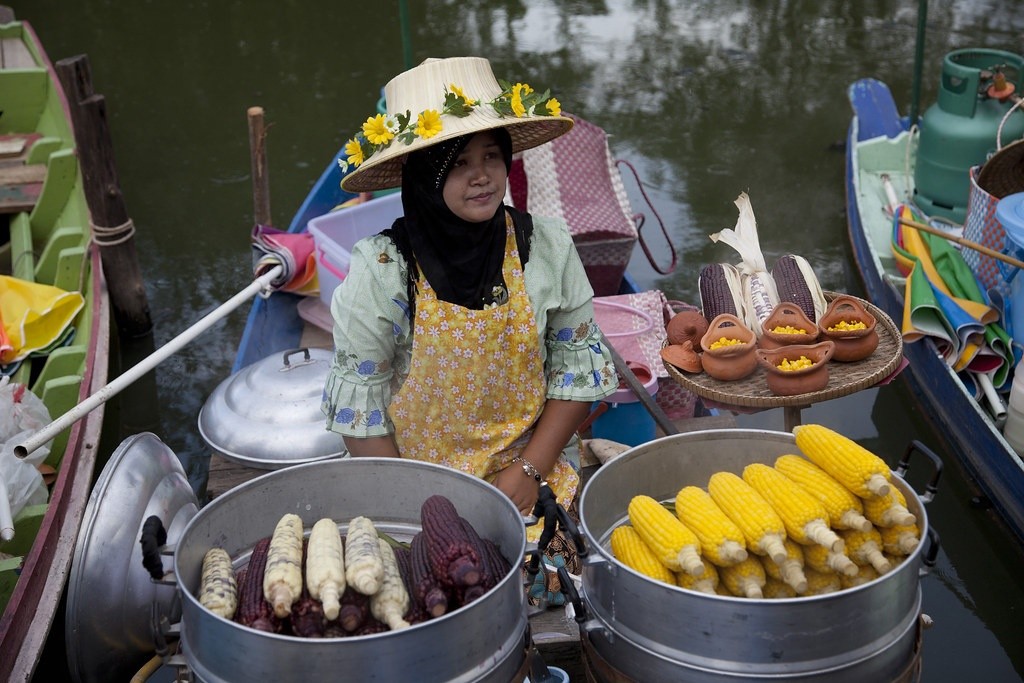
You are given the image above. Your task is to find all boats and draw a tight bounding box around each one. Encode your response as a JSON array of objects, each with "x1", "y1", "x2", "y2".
[
  {"x1": 0, "y1": 3, "x2": 111, "y2": 683},
  {"x1": 848, "y1": 77, "x2": 1024, "y2": 553}
]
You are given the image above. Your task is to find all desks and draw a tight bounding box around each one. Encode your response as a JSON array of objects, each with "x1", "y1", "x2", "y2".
[{"x1": 660, "y1": 289, "x2": 903, "y2": 433}]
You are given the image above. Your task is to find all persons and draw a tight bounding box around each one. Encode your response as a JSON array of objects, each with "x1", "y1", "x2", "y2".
[{"x1": 319, "y1": 56, "x2": 619, "y2": 615}]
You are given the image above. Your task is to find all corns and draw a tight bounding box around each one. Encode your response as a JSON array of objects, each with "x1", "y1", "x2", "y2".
[
  {"x1": 199, "y1": 495, "x2": 511, "y2": 637},
  {"x1": 699, "y1": 255, "x2": 815, "y2": 338},
  {"x1": 612, "y1": 423, "x2": 922, "y2": 600}
]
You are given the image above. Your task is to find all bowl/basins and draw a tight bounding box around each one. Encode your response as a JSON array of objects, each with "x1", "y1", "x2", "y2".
[
  {"x1": 702, "y1": 314, "x2": 757, "y2": 381},
  {"x1": 754, "y1": 341, "x2": 835, "y2": 396},
  {"x1": 817, "y1": 297, "x2": 878, "y2": 362},
  {"x1": 758, "y1": 302, "x2": 820, "y2": 357}
]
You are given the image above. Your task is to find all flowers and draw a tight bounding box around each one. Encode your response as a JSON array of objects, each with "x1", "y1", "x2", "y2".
[{"x1": 336, "y1": 85, "x2": 562, "y2": 173}]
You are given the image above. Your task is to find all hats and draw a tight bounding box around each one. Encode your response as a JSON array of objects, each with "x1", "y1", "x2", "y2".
[{"x1": 339, "y1": 57, "x2": 575, "y2": 193}]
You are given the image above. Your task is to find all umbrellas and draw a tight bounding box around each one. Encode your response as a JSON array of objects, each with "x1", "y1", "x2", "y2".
[
  {"x1": 13, "y1": 191, "x2": 371, "y2": 459},
  {"x1": 879, "y1": 172, "x2": 1016, "y2": 422}
]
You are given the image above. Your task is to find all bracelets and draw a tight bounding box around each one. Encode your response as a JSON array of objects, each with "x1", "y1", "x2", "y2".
[{"x1": 511, "y1": 457, "x2": 544, "y2": 483}]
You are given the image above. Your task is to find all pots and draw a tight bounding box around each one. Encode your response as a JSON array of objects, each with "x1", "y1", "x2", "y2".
[
  {"x1": 141, "y1": 457, "x2": 559, "y2": 683},
  {"x1": 558, "y1": 431, "x2": 945, "y2": 683}
]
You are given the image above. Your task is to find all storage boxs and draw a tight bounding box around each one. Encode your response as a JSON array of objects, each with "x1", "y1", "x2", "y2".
[{"x1": 307, "y1": 192, "x2": 404, "y2": 306}]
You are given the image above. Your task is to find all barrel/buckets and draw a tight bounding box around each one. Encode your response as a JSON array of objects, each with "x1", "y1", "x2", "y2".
[
  {"x1": 1004, "y1": 353, "x2": 1024, "y2": 459},
  {"x1": 577, "y1": 360, "x2": 660, "y2": 448}
]
